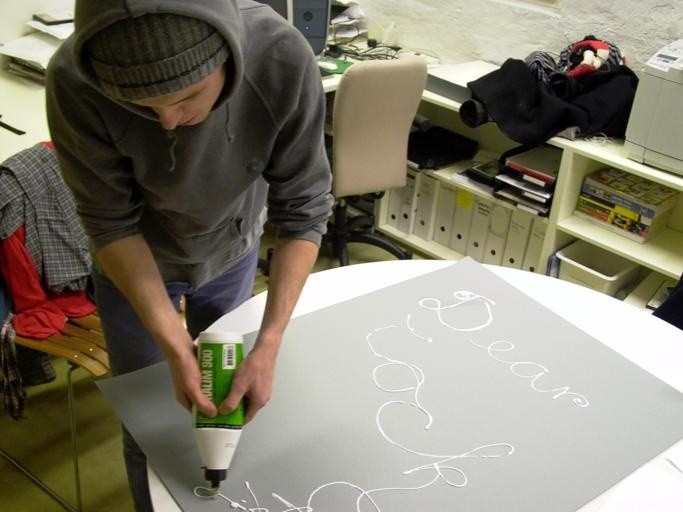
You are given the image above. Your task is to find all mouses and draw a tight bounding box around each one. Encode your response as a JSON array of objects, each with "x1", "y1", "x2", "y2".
[{"x1": 317, "y1": 61, "x2": 338, "y2": 70}]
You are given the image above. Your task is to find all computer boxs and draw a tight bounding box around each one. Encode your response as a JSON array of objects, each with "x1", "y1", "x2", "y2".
[{"x1": 255, "y1": 0, "x2": 331, "y2": 56}]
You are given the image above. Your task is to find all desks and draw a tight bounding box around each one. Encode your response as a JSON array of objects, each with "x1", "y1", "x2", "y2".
[{"x1": 145, "y1": 251, "x2": 682, "y2": 512}]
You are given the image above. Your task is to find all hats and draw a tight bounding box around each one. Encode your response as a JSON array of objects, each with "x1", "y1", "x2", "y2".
[{"x1": 76, "y1": 8, "x2": 230, "y2": 103}]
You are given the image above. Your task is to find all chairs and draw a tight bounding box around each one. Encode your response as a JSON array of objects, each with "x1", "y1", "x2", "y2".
[
  {"x1": 0, "y1": 143, "x2": 112, "y2": 512},
  {"x1": 263, "y1": 56, "x2": 429, "y2": 268}
]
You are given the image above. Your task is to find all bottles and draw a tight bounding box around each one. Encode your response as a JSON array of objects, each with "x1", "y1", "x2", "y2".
[{"x1": 189, "y1": 331, "x2": 245, "y2": 489}]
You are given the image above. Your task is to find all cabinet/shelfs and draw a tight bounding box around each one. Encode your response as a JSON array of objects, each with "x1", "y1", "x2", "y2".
[{"x1": 366, "y1": 57, "x2": 682, "y2": 321}]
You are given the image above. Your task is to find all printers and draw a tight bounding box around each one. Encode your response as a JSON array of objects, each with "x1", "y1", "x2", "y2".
[{"x1": 625, "y1": 39, "x2": 682, "y2": 181}]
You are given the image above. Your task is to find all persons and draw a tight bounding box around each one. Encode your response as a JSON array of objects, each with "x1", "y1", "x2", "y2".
[{"x1": 45, "y1": 1, "x2": 334, "y2": 511}]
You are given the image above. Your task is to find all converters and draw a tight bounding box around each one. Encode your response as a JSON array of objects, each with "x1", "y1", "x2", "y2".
[{"x1": 368, "y1": 38, "x2": 377, "y2": 47}]
